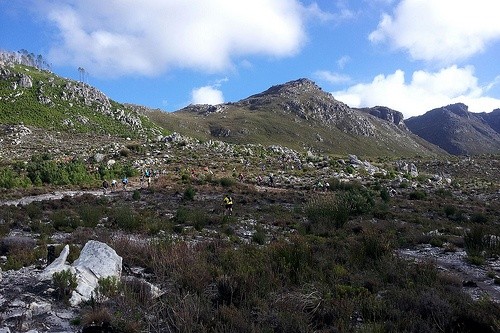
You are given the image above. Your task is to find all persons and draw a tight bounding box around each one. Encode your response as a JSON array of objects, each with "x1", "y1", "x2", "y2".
[
  {"x1": 223, "y1": 194, "x2": 233, "y2": 216},
  {"x1": 110, "y1": 171, "x2": 152, "y2": 193},
  {"x1": 103, "y1": 178, "x2": 109, "y2": 195}
]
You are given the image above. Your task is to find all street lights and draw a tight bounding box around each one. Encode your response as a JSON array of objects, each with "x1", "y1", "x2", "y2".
[{"x1": 77, "y1": 67, "x2": 85, "y2": 82}]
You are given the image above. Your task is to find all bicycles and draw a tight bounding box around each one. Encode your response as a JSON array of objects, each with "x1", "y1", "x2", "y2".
[
  {"x1": 313, "y1": 185, "x2": 332, "y2": 193},
  {"x1": 220, "y1": 204, "x2": 232, "y2": 216},
  {"x1": 102, "y1": 182, "x2": 129, "y2": 194}
]
